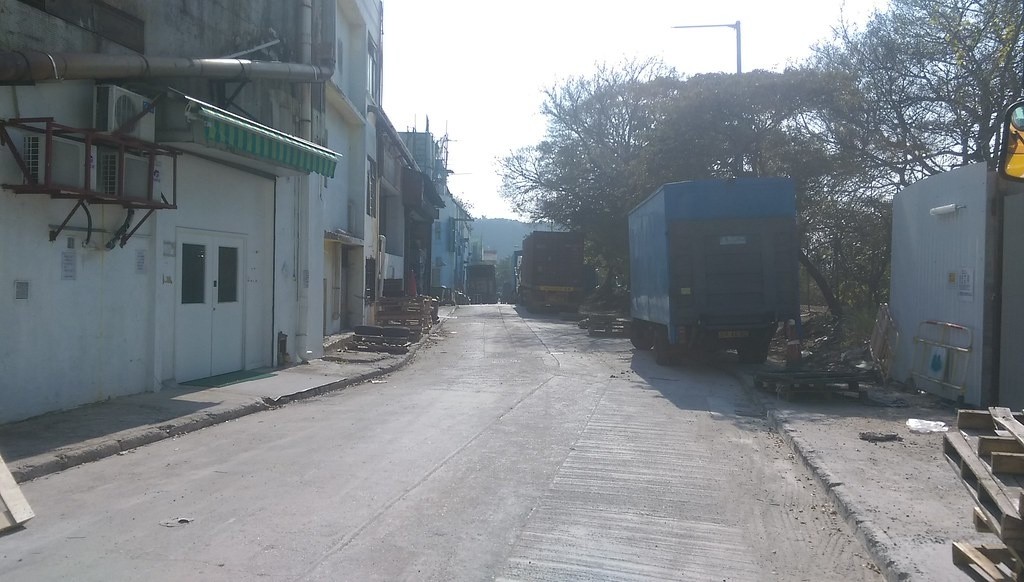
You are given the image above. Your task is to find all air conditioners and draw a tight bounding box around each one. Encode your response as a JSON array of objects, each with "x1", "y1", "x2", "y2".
[
  {"x1": 97, "y1": 147, "x2": 161, "y2": 205},
  {"x1": 23, "y1": 134, "x2": 97, "y2": 197},
  {"x1": 93, "y1": 83, "x2": 155, "y2": 143}
]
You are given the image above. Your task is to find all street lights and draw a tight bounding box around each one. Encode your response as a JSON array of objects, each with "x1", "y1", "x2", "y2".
[{"x1": 670, "y1": 20, "x2": 744, "y2": 176}]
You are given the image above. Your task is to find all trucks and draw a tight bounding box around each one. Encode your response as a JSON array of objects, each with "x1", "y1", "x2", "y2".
[
  {"x1": 624, "y1": 176, "x2": 803, "y2": 365},
  {"x1": 514, "y1": 230, "x2": 585, "y2": 313}
]
